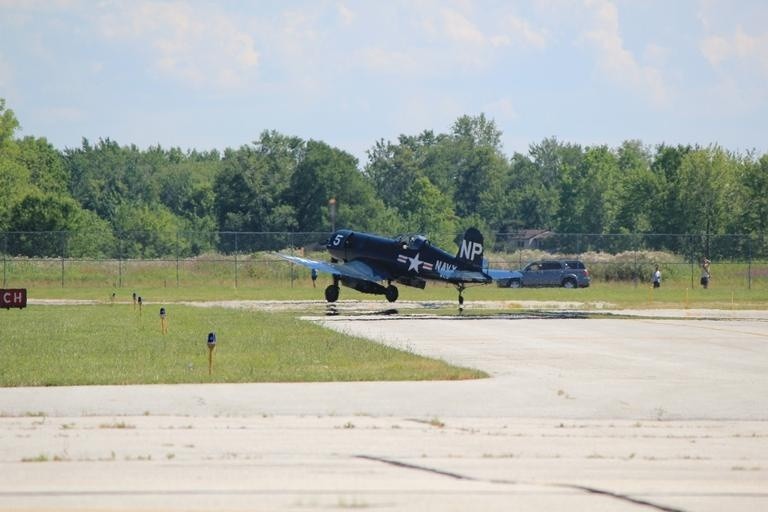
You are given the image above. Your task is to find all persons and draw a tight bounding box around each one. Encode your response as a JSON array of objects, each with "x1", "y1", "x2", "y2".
[
  {"x1": 699, "y1": 257, "x2": 711, "y2": 289},
  {"x1": 652, "y1": 266, "x2": 661, "y2": 288}
]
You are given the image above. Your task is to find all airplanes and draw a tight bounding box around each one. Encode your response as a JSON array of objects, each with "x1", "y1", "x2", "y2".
[{"x1": 273, "y1": 224, "x2": 523, "y2": 306}]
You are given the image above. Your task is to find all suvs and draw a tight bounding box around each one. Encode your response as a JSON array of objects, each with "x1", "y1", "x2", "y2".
[{"x1": 496, "y1": 257, "x2": 590, "y2": 291}]
